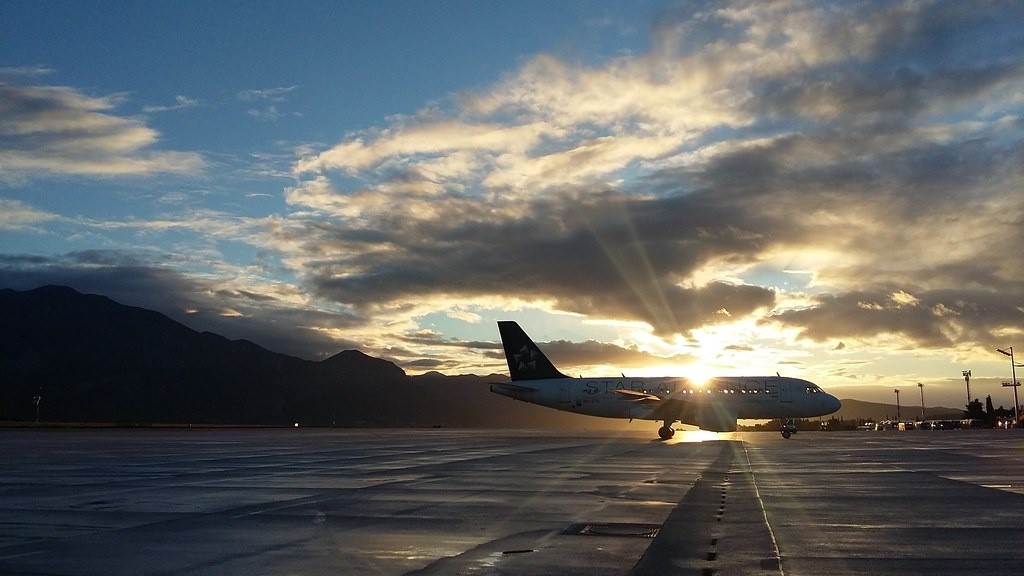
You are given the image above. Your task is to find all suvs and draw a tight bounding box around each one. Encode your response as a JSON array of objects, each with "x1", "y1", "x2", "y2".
[{"x1": 931, "y1": 420, "x2": 945, "y2": 433}]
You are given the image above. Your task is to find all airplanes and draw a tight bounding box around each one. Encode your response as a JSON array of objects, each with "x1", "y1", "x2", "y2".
[{"x1": 488, "y1": 320, "x2": 842, "y2": 440}]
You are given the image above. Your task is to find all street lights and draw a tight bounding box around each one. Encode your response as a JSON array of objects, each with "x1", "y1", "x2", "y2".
[
  {"x1": 895, "y1": 389, "x2": 900, "y2": 421},
  {"x1": 918, "y1": 382, "x2": 925, "y2": 416}
]
